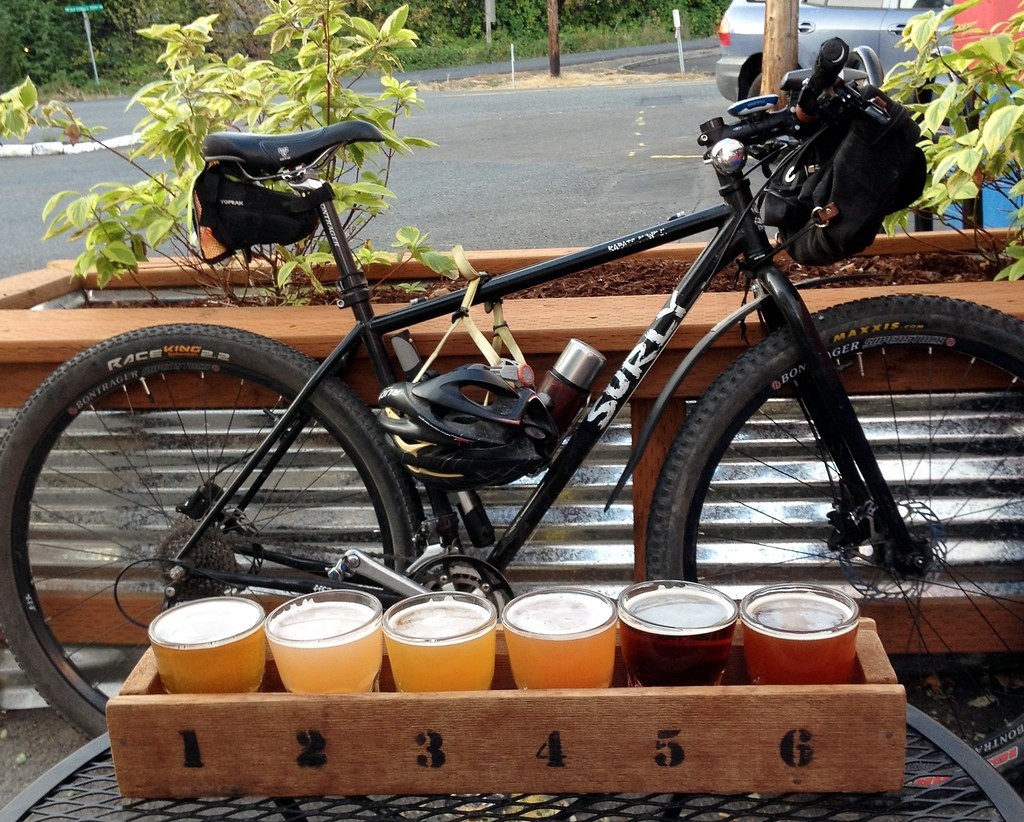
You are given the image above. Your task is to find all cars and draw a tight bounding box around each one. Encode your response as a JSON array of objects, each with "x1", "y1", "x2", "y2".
[{"x1": 710, "y1": 0, "x2": 1024, "y2": 132}]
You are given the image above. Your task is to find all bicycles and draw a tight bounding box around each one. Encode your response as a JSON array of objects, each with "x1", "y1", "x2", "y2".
[{"x1": 1, "y1": 37, "x2": 1024, "y2": 806}]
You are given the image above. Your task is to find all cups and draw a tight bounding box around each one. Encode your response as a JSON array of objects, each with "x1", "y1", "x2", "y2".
[
  {"x1": 382, "y1": 590, "x2": 497, "y2": 690},
  {"x1": 147, "y1": 596, "x2": 266, "y2": 694},
  {"x1": 501, "y1": 587, "x2": 618, "y2": 688},
  {"x1": 263, "y1": 591, "x2": 382, "y2": 693},
  {"x1": 738, "y1": 582, "x2": 860, "y2": 685},
  {"x1": 617, "y1": 579, "x2": 739, "y2": 687}
]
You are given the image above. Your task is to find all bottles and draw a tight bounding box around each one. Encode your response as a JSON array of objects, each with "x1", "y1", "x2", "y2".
[{"x1": 538, "y1": 338, "x2": 607, "y2": 435}]
